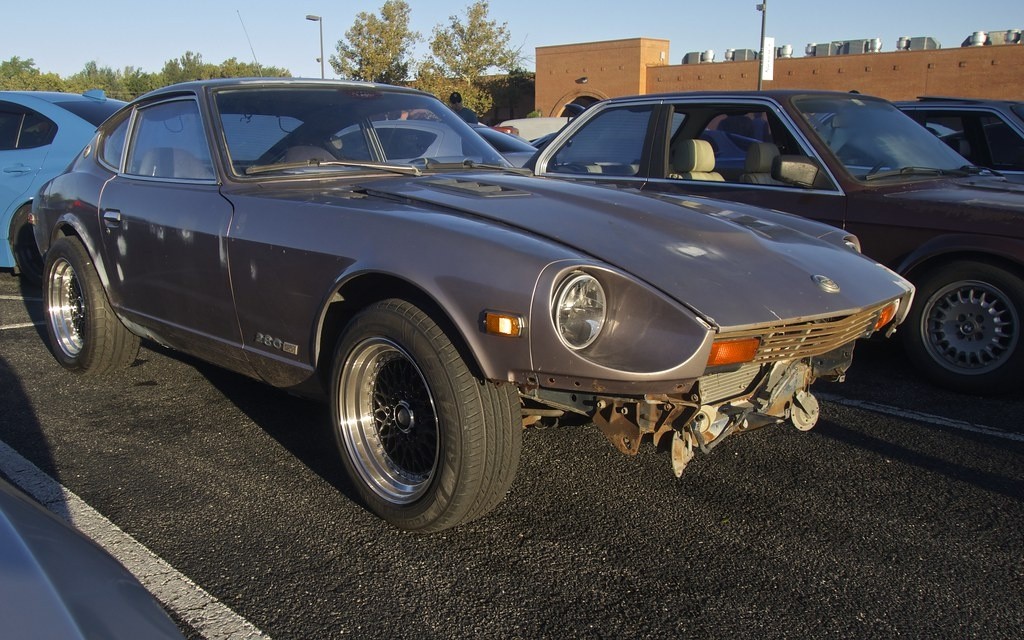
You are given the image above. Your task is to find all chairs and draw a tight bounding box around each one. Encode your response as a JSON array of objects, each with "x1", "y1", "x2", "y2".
[
  {"x1": 740, "y1": 142, "x2": 793, "y2": 187},
  {"x1": 286, "y1": 126, "x2": 339, "y2": 165},
  {"x1": 952, "y1": 137, "x2": 973, "y2": 157},
  {"x1": 139, "y1": 148, "x2": 215, "y2": 179},
  {"x1": 669, "y1": 138, "x2": 726, "y2": 182}
]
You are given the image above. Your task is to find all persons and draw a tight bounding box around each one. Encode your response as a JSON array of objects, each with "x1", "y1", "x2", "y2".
[
  {"x1": 449, "y1": 90, "x2": 477, "y2": 123},
  {"x1": 753, "y1": 112, "x2": 770, "y2": 135}
]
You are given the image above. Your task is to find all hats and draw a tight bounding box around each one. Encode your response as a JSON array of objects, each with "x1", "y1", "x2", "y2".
[{"x1": 450, "y1": 92, "x2": 462, "y2": 101}]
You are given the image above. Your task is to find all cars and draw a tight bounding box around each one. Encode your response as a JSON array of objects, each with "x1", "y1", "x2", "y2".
[
  {"x1": 492, "y1": 104, "x2": 586, "y2": 146},
  {"x1": 524, "y1": 93, "x2": 1024, "y2": 395},
  {"x1": 0, "y1": 90, "x2": 207, "y2": 273},
  {"x1": 788, "y1": 98, "x2": 1024, "y2": 183},
  {"x1": 329, "y1": 118, "x2": 539, "y2": 167}
]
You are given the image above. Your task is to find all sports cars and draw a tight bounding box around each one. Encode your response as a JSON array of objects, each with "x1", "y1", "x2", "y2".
[{"x1": 29, "y1": 76, "x2": 916, "y2": 535}]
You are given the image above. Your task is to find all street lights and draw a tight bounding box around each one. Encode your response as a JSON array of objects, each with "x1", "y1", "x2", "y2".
[
  {"x1": 756, "y1": 3, "x2": 767, "y2": 91},
  {"x1": 306, "y1": 16, "x2": 325, "y2": 79}
]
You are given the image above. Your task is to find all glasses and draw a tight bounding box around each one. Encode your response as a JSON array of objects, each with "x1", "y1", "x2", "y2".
[{"x1": 451, "y1": 99, "x2": 461, "y2": 104}]
